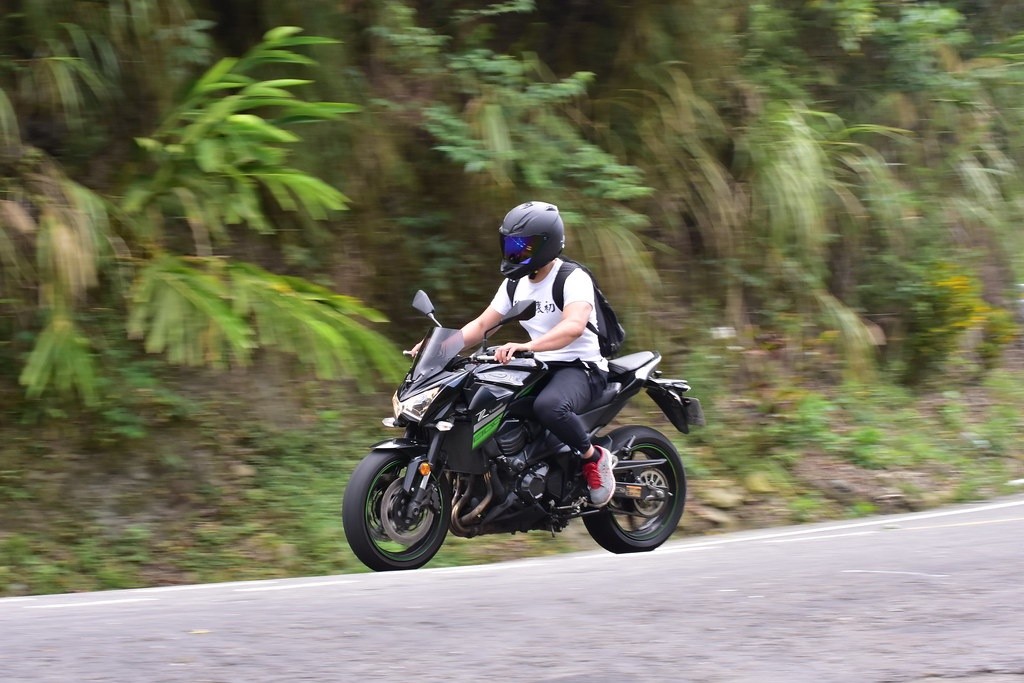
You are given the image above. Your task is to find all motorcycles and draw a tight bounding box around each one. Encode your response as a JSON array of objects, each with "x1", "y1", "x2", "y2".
[{"x1": 341, "y1": 290, "x2": 707, "y2": 572}]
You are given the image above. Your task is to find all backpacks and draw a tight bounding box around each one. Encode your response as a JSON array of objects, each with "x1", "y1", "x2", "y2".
[{"x1": 506, "y1": 248, "x2": 626, "y2": 358}]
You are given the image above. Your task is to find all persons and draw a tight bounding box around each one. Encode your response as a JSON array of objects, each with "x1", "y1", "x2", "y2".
[{"x1": 411, "y1": 201, "x2": 616, "y2": 508}]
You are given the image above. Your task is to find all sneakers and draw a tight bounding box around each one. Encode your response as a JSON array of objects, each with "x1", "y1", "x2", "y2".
[{"x1": 580, "y1": 444, "x2": 616, "y2": 507}]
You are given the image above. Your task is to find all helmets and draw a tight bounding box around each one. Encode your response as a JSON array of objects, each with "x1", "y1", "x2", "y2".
[{"x1": 499, "y1": 201, "x2": 566, "y2": 278}]
